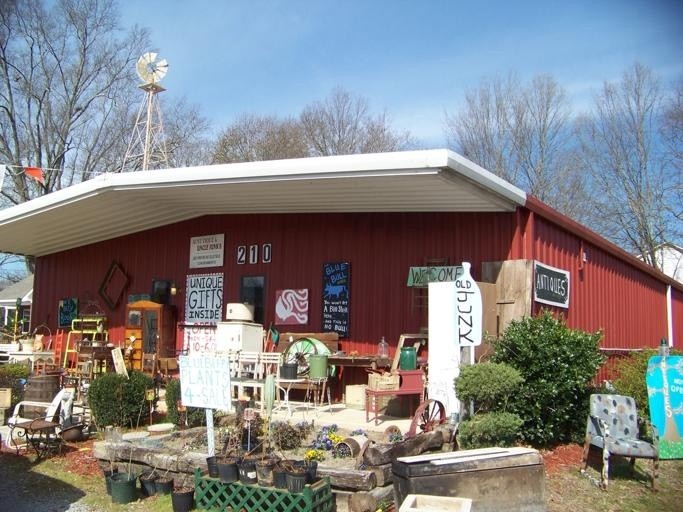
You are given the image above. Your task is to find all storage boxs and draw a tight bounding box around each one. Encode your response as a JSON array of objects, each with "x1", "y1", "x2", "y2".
[{"x1": 391, "y1": 448, "x2": 545, "y2": 512}]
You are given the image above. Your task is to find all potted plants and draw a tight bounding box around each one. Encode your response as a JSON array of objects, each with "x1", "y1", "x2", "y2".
[
  {"x1": 95, "y1": 399, "x2": 201, "y2": 511},
  {"x1": 206, "y1": 412, "x2": 317, "y2": 493}
]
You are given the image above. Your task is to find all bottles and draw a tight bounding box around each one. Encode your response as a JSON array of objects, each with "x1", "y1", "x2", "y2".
[
  {"x1": 104, "y1": 425, "x2": 113, "y2": 443},
  {"x1": 377, "y1": 336, "x2": 389, "y2": 359},
  {"x1": 112, "y1": 426, "x2": 121, "y2": 443}
]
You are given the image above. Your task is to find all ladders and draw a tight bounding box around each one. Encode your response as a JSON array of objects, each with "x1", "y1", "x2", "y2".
[{"x1": 52, "y1": 329, "x2": 64, "y2": 370}]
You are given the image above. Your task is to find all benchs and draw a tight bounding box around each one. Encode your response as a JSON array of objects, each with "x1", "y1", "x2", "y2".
[{"x1": 274, "y1": 332, "x2": 339, "y2": 405}]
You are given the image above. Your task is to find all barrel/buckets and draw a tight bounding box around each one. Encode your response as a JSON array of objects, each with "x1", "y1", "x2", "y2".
[
  {"x1": 309, "y1": 353, "x2": 328, "y2": 379},
  {"x1": 25, "y1": 374, "x2": 60, "y2": 419},
  {"x1": 282, "y1": 362, "x2": 298, "y2": 380},
  {"x1": 400, "y1": 346, "x2": 417, "y2": 371}
]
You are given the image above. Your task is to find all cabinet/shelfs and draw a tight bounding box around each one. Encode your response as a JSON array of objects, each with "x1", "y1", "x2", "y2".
[{"x1": 124, "y1": 300, "x2": 176, "y2": 369}]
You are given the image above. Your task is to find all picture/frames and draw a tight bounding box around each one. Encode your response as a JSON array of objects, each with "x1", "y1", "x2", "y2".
[{"x1": 98, "y1": 261, "x2": 129, "y2": 310}]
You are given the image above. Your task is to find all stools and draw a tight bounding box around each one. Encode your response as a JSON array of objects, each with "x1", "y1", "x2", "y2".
[{"x1": 7, "y1": 412, "x2": 66, "y2": 465}]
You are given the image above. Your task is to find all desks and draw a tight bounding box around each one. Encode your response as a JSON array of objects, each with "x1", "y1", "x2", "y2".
[
  {"x1": 78, "y1": 347, "x2": 124, "y2": 380},
  {"x1": 8, "y1": 351, "x2": 56, "y2": 372},
  {"x1": 328, "y1": 358, "x2": 389, "y2": 404},
  {"x1": 272, "y1": 378, "x2": 305, "y2": 417}
]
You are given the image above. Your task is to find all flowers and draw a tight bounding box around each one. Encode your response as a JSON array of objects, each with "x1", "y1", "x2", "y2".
[{"x1": 304, "y1": 423, "x2": 368, "y2": 466}]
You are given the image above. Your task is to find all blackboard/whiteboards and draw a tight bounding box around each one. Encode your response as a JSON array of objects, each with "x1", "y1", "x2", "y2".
[{"x1": 320, "y1": 260, "x2": 350, "y2": 337}]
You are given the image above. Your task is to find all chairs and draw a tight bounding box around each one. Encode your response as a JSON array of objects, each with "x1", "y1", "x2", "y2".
[
  {"x1": 579, "y1": 394, "x2": 659, "y2": 492},
  {"x1": 365, "y1": 370, "x2": 424, "y2": 426},
  {"x1": 228, "y1": 351, "x2": 282, "y2": 413},
  {"x1": 4, "y1": 389, "x2": 66, "y2": 450}
]
